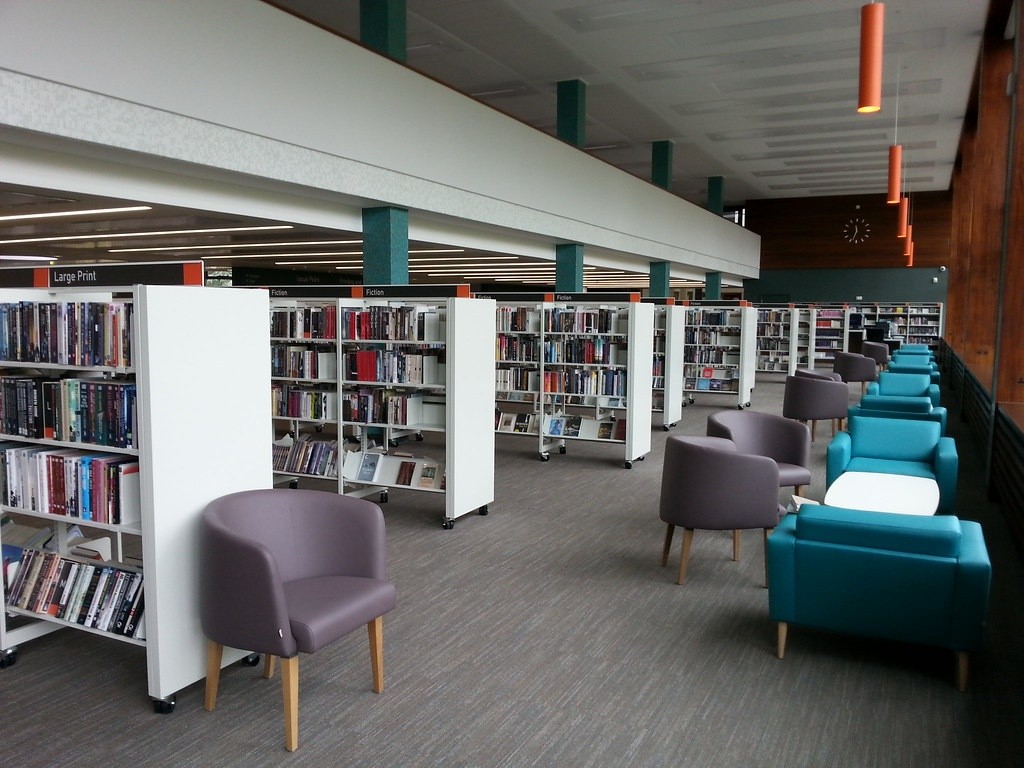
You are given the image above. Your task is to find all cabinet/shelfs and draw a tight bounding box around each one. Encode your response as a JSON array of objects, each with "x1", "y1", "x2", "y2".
[
  {"x1": 848, "y1": 305, "x2": 879, "y2": 332},
  {"x1": 269, "y1": 295, "x2": 498, "y2": 530},
  {"x1": 909, "y1": 303, "x2": 943, "y2": 345},
  {"x1": 797, "y1": 308, "x2": 817, "y2": 371},
  {"x1": 682, "y1": 305, "x2": 758, "y2": 410},
  {"x1": 755, "y1": 308, "x2": 800, "y2": 377},
  {"x1": 651, "y1": 305, "x2": 685, "y2": 431},
  {"x1": 496, "y1": 301, "x2": 655, "y2": 470},
  {"x1": 0, "y1": 282, "x2": 278, "y2": 715},
  {"x1": 878, "y1": 305, "x2": 909, "y2": 341},
  {"x1": 815, "y1": 309, "x2": 850, "y2": 360}
]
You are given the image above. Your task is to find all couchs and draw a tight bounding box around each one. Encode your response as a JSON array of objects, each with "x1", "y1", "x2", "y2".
[
  {"x1": 846, "y1": 395, "x2": 948, "y2": 437},
  {"x1": 892, "y1": 344, "x2": 934, "y2": 356},
  {"x1": 866, "y1": 372, "x2": 941, "y2": 406},
  {"x1": 824, "y1": 415, "x2": 958, "y2": 508},
  {"x1": 891, "y1": 350, "x2": 935, "y2": 361},
  {"x1": 764, "y1": 504, "x2": 992, "y2": 692},
  {"x1": 884, "y1": 364, "x2": 941, "y2": 384},
  {"x1": 887, "y1": 355, "x2": 938, "y2": 371}
]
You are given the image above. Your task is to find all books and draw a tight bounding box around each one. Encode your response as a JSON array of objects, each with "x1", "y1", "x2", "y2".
[
  {"x1": 798, "y1": 308, "x2": 940, "y2": 363},
  {"x1": 495, "y1": 304, "x2": 626, "y2": 440},
  {"x1": 0, "y1": 296, "x2": 149, "y2": 644},
  {"x1": 270, "y1": 306, "x2": 446, "y2": 489},
  {"x1": 755, "y1": 309, "x2": 790, "y2": 374},
  {"x1": 652, "y1": 309, "x2": 741, "y2": 407}
]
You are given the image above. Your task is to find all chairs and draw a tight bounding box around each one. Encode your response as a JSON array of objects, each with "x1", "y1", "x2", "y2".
[
  {"x1": 706, "y1": 408, "x2": 812, "y2": 497},
  {"x1": 783, "y1": 376, "x2": 850, "y2": 442},
  {"x1": 198, "y1": 487, "x2": 397, "y2": 753},
  {"x1": 833, "y1": 351, "x2": 877, "y2": 396},
  {"x1": 795, "y1": 369, "x2": 842, "y2": 382},
  {"x1": 659, "y1": 434, "x2": 780, "y2": 585},
  {"x1": 861, "y1": 341, "x2": 891, "y2": 372}
]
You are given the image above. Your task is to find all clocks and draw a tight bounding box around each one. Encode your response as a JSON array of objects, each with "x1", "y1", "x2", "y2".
[{"x1": 843, "y1": 218, "x2": 870, "y2": 244}]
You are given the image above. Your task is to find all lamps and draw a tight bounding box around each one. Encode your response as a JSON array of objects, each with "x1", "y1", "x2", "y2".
[
  {"x1": 886, "y1": 111, "x2": 902, "y2": 204},
  {"x1": 907, "y1": 205, "x2": 914, "y2": 267},
  {"x1": 856, "y1": 0, "x2": 885, "y2": 114},
  {"x1": 897, "y1": 160, "x2": 908, "y2": 238},
  {"x1": 903, "y1": 183, "x2": 912, "y2": 256}
]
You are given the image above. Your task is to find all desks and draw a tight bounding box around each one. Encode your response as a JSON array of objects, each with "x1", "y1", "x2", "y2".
[{"x1": 823, "y1": 471, "x2": 940, "y2": 516}]
